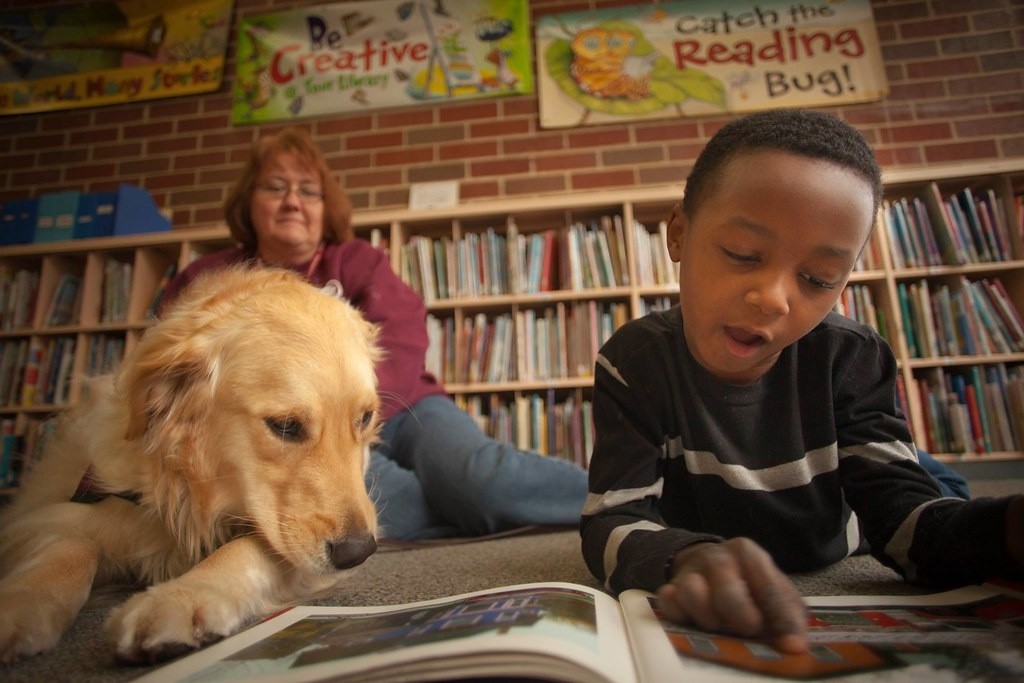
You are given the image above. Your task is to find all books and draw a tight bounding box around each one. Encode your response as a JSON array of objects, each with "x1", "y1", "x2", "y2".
[
  {"x1": 131, "y1": 583, "x2": 1024, "y2": 683},
  {"x1": 831, "y1": 274, "x2": 1024, "y2": 357},
  {"x1": 454, "y1": 387, "x2": 596, "y2": 470},
  {"x1": 0, "y1": 412, "x2": 58, "y2": 490},
  {"x1": 369, "y1": 214, "x2": 630, "y2": 302},
  {"x1": 425, "y1": 300, "x2": 631, "y2": 382},
  {"x1": 629, "y1": 215, "x2": 679, "y2": 285},
  {"x1": 0, "y1": 253, "x2": 174, "y2": 330},
  {"x1": 896, "y1": 361, "x2": 1024, "y2": 453},
  {"x1": 0, "y1": 335, "x2": 123, "y2": 408},
  {"x1": 851, "y1": 187, "x2": 1024, "y2": 271},
  {"x1": 638, "y1": 296, "x2": 671, "y2": 316}
]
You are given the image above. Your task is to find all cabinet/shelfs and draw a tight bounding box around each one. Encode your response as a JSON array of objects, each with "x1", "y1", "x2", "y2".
[
  {"x1": 343, "y1": 183, "x2": 680, "y2": 475},
  {"x1": 844, "y1": 156, "x2": 1024, "y2": 460},
  {"x1": 0, "y1": 229, "x2": 231, "y2": 501}
]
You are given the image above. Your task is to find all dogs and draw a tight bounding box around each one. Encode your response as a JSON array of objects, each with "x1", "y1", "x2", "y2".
[{"x1": 0, "y1": 268, "x2": 392, "y2": 665}]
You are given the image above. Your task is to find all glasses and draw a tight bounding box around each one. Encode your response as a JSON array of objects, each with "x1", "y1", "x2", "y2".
[{"x1": 247, "y1": 182, "x2": 326, "y2": 202}]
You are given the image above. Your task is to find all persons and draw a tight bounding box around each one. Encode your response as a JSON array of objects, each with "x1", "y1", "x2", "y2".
[
  {"x1": 149, "y1": 125, "x2": 590, "y2": 545},
  {"x1": 577, "y1": 107, "x2": 1024, "y2": 652}
]
